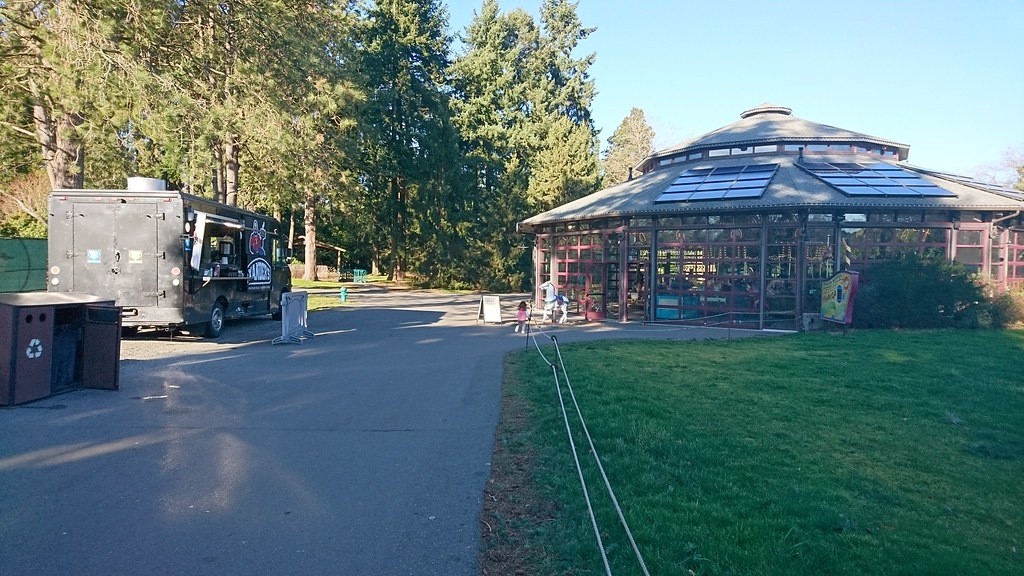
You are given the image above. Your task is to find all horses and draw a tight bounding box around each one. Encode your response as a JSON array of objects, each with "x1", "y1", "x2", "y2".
[{"x1": 539, "y1": 281, "x2": 570, "y2": 324}]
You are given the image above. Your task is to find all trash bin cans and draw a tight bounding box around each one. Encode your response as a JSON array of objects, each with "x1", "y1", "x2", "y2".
[{"x1": 0, "y1": 291, "x2": 125, "y2": 408}]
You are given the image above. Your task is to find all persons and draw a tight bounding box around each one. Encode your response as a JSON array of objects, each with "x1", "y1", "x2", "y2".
[{"x1": 514, "y1": 301, "x2": 529, "y2": 334}]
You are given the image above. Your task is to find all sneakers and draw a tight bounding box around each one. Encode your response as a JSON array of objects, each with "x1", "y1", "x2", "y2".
[
  {"x1": 521, "y1": 330, "x2": 525, "y2": 335},
  {"x1": 515, "y1": 326, "x2": 519, "y2": 333}
]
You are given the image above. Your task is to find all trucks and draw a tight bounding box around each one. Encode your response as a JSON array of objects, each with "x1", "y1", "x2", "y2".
[{"x1": 47, "y1": 178, "x2": 293, "y2": 339}]
